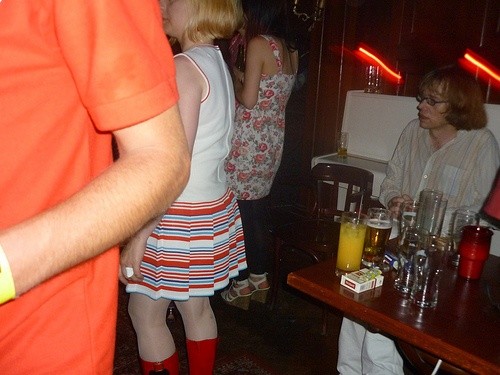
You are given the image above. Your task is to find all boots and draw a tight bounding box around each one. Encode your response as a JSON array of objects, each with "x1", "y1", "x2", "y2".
[
  {"x1": 139, "y1": 351, "x2": 178, "y2": 375},
  {"x1": 186, "y1": 334, "x2": 220, "y2": 375}
]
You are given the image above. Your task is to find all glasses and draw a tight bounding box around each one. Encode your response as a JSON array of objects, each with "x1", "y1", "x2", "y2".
[{"x1": 416, "y1": 94, "x2": 450, "y2": 106}]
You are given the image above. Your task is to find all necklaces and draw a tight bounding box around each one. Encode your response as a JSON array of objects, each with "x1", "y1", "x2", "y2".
[{"x1": 126, "y1": 267, "x2": 134, "y2": 277}]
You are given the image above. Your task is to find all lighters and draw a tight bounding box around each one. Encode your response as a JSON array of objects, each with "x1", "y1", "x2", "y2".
[{"x1": 359, "y1": 266, "x2": 384, "y2": 281}]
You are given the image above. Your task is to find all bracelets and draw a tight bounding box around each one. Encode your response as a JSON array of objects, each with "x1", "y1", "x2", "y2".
[{"x1": 0, "y1": 246, "x2": 16, "y2": 304}]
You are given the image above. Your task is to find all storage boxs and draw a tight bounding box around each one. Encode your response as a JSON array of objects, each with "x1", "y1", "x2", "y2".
[{"x1": 340, "y1": 268, "x2": 384, "y2": 293}]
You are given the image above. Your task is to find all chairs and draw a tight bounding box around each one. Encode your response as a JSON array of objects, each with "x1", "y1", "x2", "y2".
[{"x1": 267, "y1": 164, "x2": 374, "y2": 337}]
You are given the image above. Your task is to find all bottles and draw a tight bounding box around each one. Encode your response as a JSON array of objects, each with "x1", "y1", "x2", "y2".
[{"x1": 236, "y1": 44, "x2": 245, "y2": 73}]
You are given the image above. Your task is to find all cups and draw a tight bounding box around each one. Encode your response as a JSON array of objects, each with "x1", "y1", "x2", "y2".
[
  {"x1": 365, "y1": 65, "x2": 379, "y2": 94},
  {"x1": 335, "y1": 212, "x2": 369, "y2": 272},
  {"x1": 362, "y1": 207, "x2": 393, "y2": 265},
  {"x1": 393, "y1": 188, "x2": 493, "y2": 310},
  {"x1": 337, "y1": 132, "x2": 349, "y2": 159}
]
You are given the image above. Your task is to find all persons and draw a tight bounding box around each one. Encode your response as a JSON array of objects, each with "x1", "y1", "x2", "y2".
[
  {"x1": 0, "y1": 0, "x2": 191, "y2": 375},
  {"x1": 120, "y1": 0, "x2": 248, "y2": 375},
  {"x1": 337, "y1": 67, "x2": 500, "y2": 374},
  {"x1": 220, "y1": 0, "x2": 300, "y2": 308}
]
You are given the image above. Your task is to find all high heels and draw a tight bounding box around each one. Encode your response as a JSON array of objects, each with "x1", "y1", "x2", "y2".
[
  {"x1": 247, "y1": 272, "x2": 270, "y2": 304},
  {"x1": 221, "y1": 279, "x2": 252, "y2": 310}
]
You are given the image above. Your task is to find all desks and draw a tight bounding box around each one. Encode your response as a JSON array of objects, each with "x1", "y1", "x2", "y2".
[
  {"x1": 287, "y1": 249, "x2": 500, "y2": 375},
  {"x1": 311, "y1": 153, "x2": 389, "y2": 222}
]
